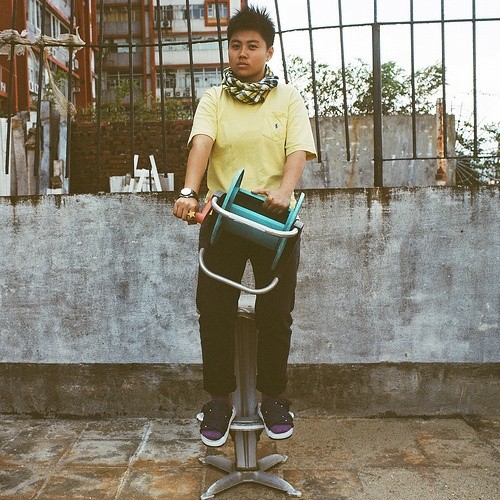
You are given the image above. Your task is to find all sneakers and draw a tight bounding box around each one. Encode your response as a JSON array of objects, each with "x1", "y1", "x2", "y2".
[
  {"x1": 199, "y1": 401, "x2": 237, "y2": 446},
  {"x1": 257, "y1": 398, "x2": 294, "y2": 441}
]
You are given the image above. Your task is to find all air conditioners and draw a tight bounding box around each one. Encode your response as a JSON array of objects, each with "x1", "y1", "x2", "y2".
[
  {"x1": 164, "y1": 91, "x2": 174, "y2": 98},
  {"x1": 160, "y1": 20, "x2": 171, "y2": 29},
  {"x1": 175, "y1": 91, "x2": 184, "y2": 97},
  {"x1": 184, "y1": 86, "x2": 193, "y2": 97}
]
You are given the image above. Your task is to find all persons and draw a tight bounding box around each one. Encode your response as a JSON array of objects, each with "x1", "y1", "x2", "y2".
[{"x1": 172, "y1": 6, "x2": 318, "y2": 446}]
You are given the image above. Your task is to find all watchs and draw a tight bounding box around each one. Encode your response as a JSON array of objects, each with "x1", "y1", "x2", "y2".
[{"x1": 178, "y1": 188, "x2": 200, "y2": 202}]
